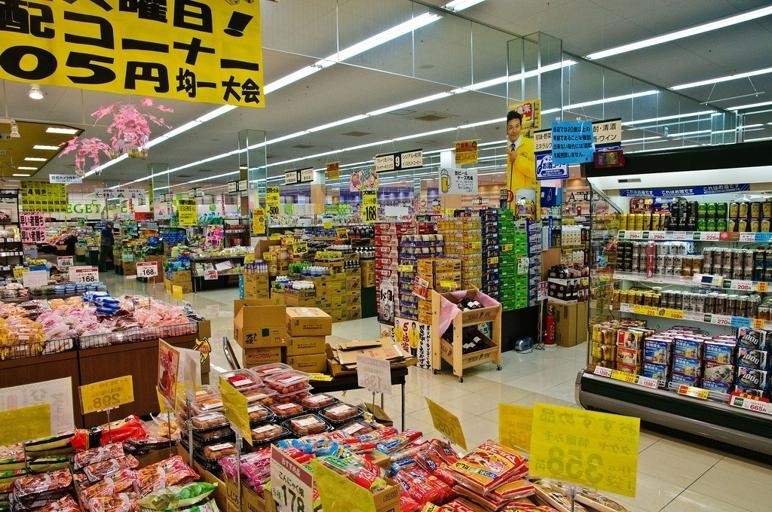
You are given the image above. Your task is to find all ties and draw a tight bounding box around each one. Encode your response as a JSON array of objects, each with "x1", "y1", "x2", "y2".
[{"x1": 510, "y1": 142, "x2": 516, "y2": 153}]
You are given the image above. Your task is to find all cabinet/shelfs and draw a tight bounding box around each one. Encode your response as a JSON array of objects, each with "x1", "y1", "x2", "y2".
[{"x1": 1, "y1": 222, "x2": 24, "y2": 277}]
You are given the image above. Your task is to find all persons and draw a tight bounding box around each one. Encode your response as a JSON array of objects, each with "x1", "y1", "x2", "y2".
[
  {"x1": 385, "y1": 289, "x2": 394, "y2": 307},
  {"x1": 64, "y1": 230, "x2": 78, "y2": 256},
  {"x1": 99, "y1": 222, "x2": 114, "y2": 272},
  {"x1": 506, "y1": 110, "x2": 535, "y2": 216},
  {"x1": 395, "y1": 320, "x2": 420, "y2": 367}
]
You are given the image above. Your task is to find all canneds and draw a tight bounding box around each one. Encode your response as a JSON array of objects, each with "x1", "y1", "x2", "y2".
[{"x1": 612, "y1": 198, "x2": 772, "y2": 321}]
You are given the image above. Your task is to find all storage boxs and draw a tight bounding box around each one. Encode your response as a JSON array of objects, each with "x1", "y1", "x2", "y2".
[
  {"x1": 120, "y1": 255, "x2": 193, "y2": 293},
  {"x1": 232, "y1": 209, "x2": 541, "y2": 373}
]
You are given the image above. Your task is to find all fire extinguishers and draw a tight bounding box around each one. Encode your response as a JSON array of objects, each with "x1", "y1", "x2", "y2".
[{"x1": 545, "y1": 305, "x2": 556, "y2": 344}]
